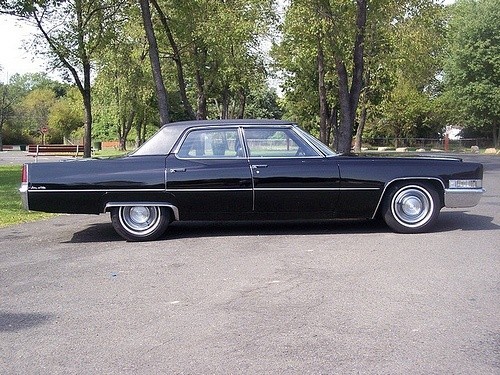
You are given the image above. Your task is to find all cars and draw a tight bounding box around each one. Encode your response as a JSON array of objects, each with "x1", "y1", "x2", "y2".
[{"x1": 18, "y1": 116, "x2": 486, "y2": 234}]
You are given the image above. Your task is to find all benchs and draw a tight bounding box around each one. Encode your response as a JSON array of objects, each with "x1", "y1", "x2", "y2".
[{"x1": 26, "y1": 144, "x2": 94, "y2": 158}]
